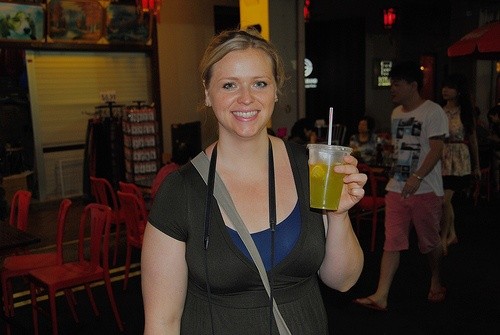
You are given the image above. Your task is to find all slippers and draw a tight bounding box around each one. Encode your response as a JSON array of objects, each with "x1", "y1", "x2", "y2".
[
  {"x1": 427, "y1": 285, "x2": 447, "y2": 303},
  {"x1": 351, "y1": 297, "x2": 389, "y2": 311}
]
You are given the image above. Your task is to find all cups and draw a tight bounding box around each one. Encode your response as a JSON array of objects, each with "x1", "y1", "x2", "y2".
[{"x1": 308, "y1": 143, "x2": 352, "y2": 210}]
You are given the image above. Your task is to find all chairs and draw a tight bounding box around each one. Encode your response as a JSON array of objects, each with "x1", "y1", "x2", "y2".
[
  {"x1": 27, "y1": 203, "x2": 124, "y2": 335},
  {"x1": 1, "y1": 198, "x2": 72, "y2": 318},
  {"x1": 90, "y1": 175, "x2": 125, "y2": 267},
  {"x1": 9, "y1": 189, "x2": 31, "y2": 231},
  {"x1": 116, "y1": 190, "x2": 147, "y2": 280},
  {"x1": 358, "y1": 164, "x2": 385, "y2": 251},
  {"x1": 118, "y1": 181, "x2": 144, "y2": 205}
]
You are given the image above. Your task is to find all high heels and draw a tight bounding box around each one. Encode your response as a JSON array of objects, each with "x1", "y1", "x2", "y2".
[
  {"x1": 449, "y1": 236, "x2": 457, "y2": 245},
  {"x1": 441, "y1": 251, "x2": 448, "y2": 256}
]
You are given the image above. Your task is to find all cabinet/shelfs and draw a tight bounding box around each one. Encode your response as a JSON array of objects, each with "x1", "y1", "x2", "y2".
[{"x1": 80, "y1": 100, "x2": 163, "y2": 197}]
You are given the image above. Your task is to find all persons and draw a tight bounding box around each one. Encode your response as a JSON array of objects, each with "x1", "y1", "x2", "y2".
[
  {"x1": 285, "y1": 118, "x2": 314, "y2": 145},
  {"x1": 350, "y1": 116, "x2": 386, "y2": 163},
  {"x1": 141, "y1": 30, "x2": 368, "y2": 335},
  {"x1": 354, "y1": 63, "x2": 450, "y2": 310},
  {"x1": 438, "y1": 75, "x2": 482, "y2": 256}
]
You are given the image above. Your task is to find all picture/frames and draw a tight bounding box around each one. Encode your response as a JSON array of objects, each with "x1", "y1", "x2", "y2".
[{"x1": 0, "y1": 0, "x2": 155, "y2": 49}]
